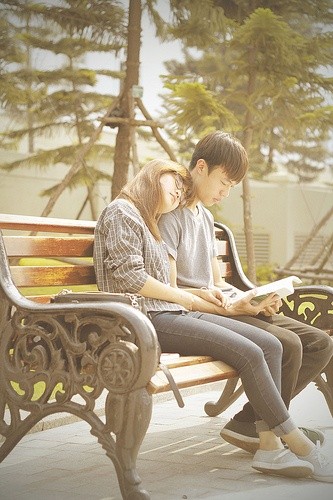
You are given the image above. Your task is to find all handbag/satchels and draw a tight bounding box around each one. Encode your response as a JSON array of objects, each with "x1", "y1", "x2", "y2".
[{"x1": 31, "y1": 288, "x2": 161, "y2": 373}]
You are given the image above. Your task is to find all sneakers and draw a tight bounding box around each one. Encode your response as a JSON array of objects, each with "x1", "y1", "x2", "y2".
[
  {"x1": 220, "y1": 418, "x2": 326, "y2": 453},
  {"x1": 296, "y1": 445, "x2": 333, "y2": 482},
  {"x1": 252, "y1": 446, "x2": 314, "y2": 478}
]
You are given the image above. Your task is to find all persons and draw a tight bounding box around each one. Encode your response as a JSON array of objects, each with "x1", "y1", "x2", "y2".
[
  {"x1": 152, "y1": 130, "x2": 333, "y2": 456},
  {"x1": 92, "y1": 159, "x2": 333, "y2": 481}
]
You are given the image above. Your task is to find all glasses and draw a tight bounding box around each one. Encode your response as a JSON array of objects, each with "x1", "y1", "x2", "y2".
[{"x1": 172, "y1": 173, "x2": 194, "y2": 200}]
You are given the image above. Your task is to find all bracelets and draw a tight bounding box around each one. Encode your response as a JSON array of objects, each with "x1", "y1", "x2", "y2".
[{"x1": 199, "y1": 286, "x2": 208, "y2": 298}]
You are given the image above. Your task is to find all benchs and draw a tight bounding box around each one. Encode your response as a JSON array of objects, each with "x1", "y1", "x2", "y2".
[{"x1": 0, "y1": 213, "x2": 333, "y2": 500}]
